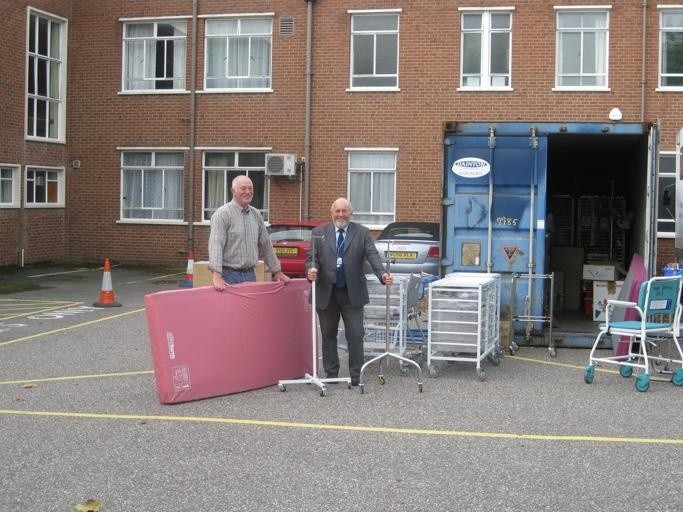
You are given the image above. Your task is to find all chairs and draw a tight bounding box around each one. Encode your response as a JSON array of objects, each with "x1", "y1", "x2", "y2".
[{"x1": 583, "y1": 276, "x2": 683, "y2": 391}]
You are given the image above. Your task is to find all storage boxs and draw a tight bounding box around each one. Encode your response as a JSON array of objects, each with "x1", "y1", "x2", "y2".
[{"x1": 544, "y1": 247, "x2": 624, "y2": 321}]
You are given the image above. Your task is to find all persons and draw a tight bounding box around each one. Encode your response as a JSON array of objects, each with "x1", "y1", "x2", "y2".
[
  {"x1": 207, "y1": 174, "x2": 290, "y2": 291},
  {"x1": 303, "y1": 197, "x2": 392, "y2": 386}
]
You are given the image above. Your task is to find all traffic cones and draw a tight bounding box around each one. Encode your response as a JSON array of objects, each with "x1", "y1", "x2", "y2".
[
  {"x1": 93, "y1": 256, "x2": 122, "y2": 307},
  {"x1": 179, "y1": 249, "x2": 194, "y2": 287}
]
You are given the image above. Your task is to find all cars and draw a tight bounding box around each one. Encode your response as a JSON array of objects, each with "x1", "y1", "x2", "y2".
[{"x1": 266, "y1": 222, "x2": 439, "y2": 281}]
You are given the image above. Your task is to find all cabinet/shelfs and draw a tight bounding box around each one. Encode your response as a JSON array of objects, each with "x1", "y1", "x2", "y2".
[
  {"x1": 363, "y1": 276, "x2": 407, "y2": 357},
  {"x1": 428, "y1": 272, "x2": 501, "y2": 369}
]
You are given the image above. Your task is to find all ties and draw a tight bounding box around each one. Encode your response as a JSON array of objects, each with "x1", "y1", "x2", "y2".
[{"x1": 336, "y1": 229, "x2": 346, "y2": 288}]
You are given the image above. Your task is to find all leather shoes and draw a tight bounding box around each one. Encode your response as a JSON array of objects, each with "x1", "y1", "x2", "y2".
[
  {"x1": 351, "y1": 376, "x2": 360, "y2": 386},
  {"x1": 326, "y1": 373, "x2": 338, "y2": 382}
]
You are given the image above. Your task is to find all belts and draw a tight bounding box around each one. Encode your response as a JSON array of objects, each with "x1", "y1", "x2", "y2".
[{"x1": 224, "y1": 266, "x2": 253, "y2": 273}]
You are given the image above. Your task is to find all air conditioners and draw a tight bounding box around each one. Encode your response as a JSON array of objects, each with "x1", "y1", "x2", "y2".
[{"x1": 265, "y1": 153, "x2": 295, "y2": 176}]
